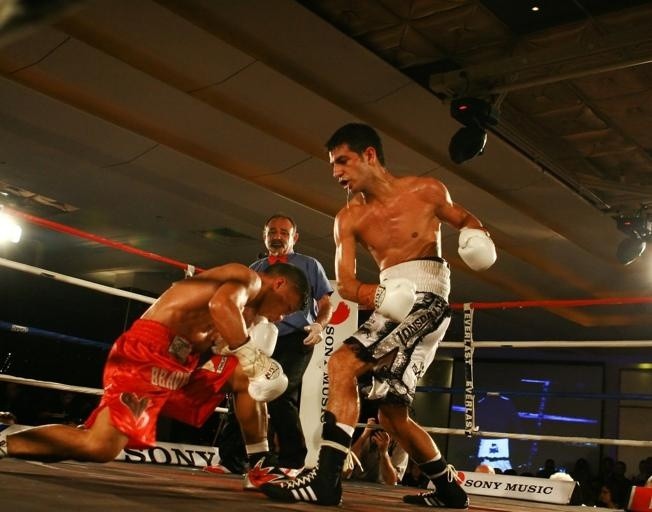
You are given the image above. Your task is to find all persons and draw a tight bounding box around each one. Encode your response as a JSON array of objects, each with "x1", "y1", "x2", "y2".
[
  {"x1": 403, "y1": 453, "x2": 652, "y2": 512},
  {"x1": 1, "y1": 257, "x2": 312, "y2": 493},
  {"x1": 343, "y1": 406, "x2": 414, "y2": 487},
  {"x1": 259, "y1": 120, "x2": 502, "y2": 511},
  {"x1": 199, "y1": 211, "x2": 335, "y2": 482}
]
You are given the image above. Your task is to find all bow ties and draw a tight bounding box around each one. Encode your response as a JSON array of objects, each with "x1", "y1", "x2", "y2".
[{"x1": 266, "y1": 254, "x2": 289, "y2": 265}]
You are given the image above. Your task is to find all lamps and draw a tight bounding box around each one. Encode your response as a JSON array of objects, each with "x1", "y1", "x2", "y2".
[
  {"x1": 612, "y1": 207, "x2": 651, "y2": 265},
  {"x1": 449, "y1": 92, "x2": 500, "y2": 164}
]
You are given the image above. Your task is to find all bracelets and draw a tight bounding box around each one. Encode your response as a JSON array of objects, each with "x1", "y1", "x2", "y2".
[{"x1": 357, "y1": 284, "x2": 377, "y2": 309}]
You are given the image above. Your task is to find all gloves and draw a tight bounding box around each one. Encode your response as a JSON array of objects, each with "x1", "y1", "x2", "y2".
[
  {"x1": 454, "y1": 224, "x2": 500, "y2": 271},
  {"x1": 355, "y1": 276, "x2": 418, "y2": 324},
  {"x1": 246, "y1": 314, "x2": 280, "y2": 358},
  {"x1": 227, "y1": 334, "x2": 290, "y2": 403},
  {"x1": 303, "y1": 321, "x2": 325, "y2": 346}
]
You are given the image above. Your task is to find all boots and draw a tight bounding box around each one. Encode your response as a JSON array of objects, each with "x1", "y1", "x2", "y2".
[
  {"x1": 258, "y1": 407, "x2": 354, "y2": 506},
  {"x1": 401, "y1": 455, "x2": 470, "y2": 511}
]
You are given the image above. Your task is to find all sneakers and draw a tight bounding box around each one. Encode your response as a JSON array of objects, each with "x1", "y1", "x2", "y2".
[
  {"x1": 202, "y1": 462, "x2": 236, "y2": 475},
  {"x1": 241, "y1": 449, "x2": 297, "y2": 492}
]
